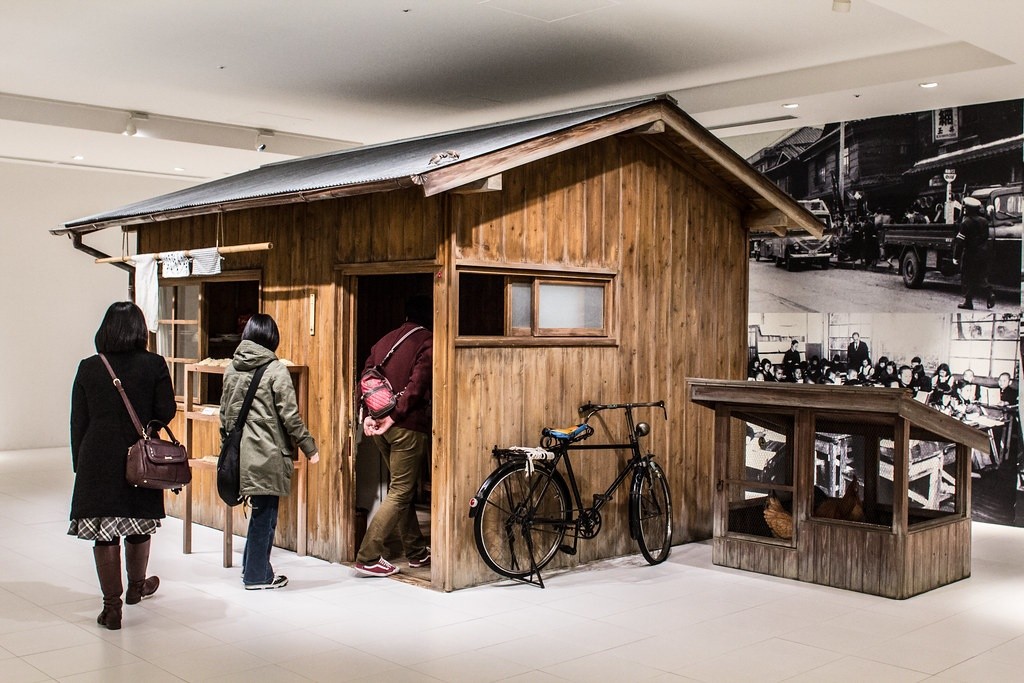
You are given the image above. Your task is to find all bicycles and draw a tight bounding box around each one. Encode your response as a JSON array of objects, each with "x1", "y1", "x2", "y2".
[{"x1": 468, "y1": 399, "x2": 674, "y2": 588}]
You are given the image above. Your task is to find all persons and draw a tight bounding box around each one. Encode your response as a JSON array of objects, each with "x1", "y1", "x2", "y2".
[
  {"x1": 952, "y1": 197, "x2": 996, "y2": 309},
  {"x1": 831, "y1": 205, "x2": 930, "y2": 271},
  {"x1": 997, "y1": 372, "x2": 1021, "y2": 424},
  {"x1": 67, "y1": 301, "x2": 178, "y2": 630},
  {"x1": 354, "y1": 295, "x2": 433, "y2": 576},
  {"x1": 747, "y1": 332, "x2": 991, "y2": 469},
  {"x1": 219, "y1": 313, "x2": 320, "y2": 590}
]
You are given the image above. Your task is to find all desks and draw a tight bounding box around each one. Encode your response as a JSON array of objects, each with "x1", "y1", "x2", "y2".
[{"x1": 813, "y1": 407, "x2": 1006, "y2": 510}]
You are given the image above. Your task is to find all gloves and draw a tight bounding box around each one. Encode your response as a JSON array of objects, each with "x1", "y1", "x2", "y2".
[{"x1": 953, "y1": 258, "x2": 960, "y2": 265}]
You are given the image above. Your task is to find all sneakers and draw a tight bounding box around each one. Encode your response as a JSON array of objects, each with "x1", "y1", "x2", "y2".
[
  {"x1": 354, "y1": 555, "x2": 400, "y2": 577},
  {"x1": 245, "y1": 576, "x2": 288, "y2": 590},
  {"x1": 409, "y1": 547, "x2": 431, "y2": 568}
]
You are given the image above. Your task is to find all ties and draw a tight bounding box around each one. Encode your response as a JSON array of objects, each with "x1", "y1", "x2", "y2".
[{"x1": 856, "y1": 342, "x2": 857, "y2": 349}]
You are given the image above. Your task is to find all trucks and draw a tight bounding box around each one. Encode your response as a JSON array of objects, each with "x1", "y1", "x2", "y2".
[{"x1": 753, "y1": 199, "x2": 835, "y2": 273}]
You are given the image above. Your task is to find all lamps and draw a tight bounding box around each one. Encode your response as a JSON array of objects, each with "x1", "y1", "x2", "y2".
[
  {"x1": 126, "y1": 113, "x2": 149, "y2": 136},
  {"x1": 255, "y1": 129, "x2": 274, "y2": 152}
]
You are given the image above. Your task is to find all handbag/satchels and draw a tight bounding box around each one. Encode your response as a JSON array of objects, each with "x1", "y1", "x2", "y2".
[
  {"x1": 360, "y1": 368, "x2": 396, "y2": 420},
  {"x1": 125, "y1": 420, "x2": 192, "y2": 495},
  {"x1": 216, "y1": 428, "x2": 245, "y2": 507}
]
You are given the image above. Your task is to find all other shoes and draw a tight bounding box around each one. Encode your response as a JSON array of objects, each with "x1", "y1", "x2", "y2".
[
  {"x1": 958, "y1": 303, "x2": 973, "y2": 309},
  {"x1": 987, "y1": 294, "x2": 995, "y2": 308}
]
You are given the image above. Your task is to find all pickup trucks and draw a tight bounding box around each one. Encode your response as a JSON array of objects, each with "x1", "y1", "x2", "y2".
[{"x1": 881, "y1": 183, "x2": 1021, "y2": 291}]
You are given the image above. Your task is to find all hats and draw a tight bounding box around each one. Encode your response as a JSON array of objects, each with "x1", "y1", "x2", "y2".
[{"x1": 963, "y1": 196, "x2": 981, "y2": 207}]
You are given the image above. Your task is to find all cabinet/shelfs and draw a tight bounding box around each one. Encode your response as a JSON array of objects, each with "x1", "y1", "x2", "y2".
[{"x1": 179, "y1": 363, "x2": 310, "y2": 568}]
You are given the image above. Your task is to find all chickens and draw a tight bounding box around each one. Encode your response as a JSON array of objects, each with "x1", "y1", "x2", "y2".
[
  {"x1": 761, "y1": 495, "x2": 793, "y2": 541},
  {"x1": 814, "y1": 473, "x2": 866, "y2": 524}
]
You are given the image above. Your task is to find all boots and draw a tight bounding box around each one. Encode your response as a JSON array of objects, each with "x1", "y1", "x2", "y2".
[
  {"x1": 93, "y1": 545, "x2": 123, "y2": 630},
  {"x1": 124, "y1": 536, "x2": 159, "y2": 605}
]
